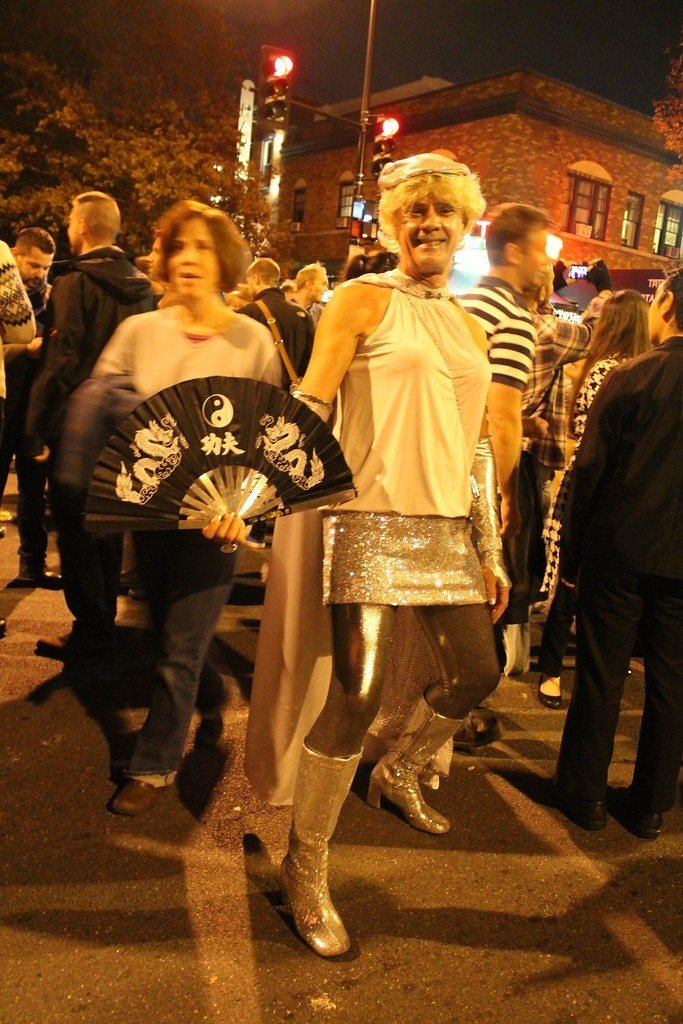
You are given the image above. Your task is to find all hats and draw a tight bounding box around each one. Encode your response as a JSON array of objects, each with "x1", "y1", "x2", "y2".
[{"x1": 378, "y1": 152, "x2": 471, "y2": 189}]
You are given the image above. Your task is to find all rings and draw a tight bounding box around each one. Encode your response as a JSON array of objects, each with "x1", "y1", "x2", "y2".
[{"x1": 219, "y1": 542, "x2": 237, "y2": 553}]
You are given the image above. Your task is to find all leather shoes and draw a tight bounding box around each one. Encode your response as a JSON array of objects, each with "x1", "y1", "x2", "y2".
[
  {"x1": 111, "y1": 780, "x2": 164, "y2": 815},
  {"x1": 196, "y1": 709, "x2": 224, "y2": 746},
  {"x1": 537, "y1": 777, "x2": 608, "y2": 832},
  {"x1": 606, "y1": 786, "x2": 664, "y2": 838}
]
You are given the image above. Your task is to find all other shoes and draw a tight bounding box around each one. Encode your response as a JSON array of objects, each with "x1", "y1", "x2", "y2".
[
  {"x1": 35, "y1": 630, "x2": 107, "y2": 653},
  {"x1": 538, "y1": 674, "x2": 562, "y2": 709},
  {"x1": 18, "y1": 569, "x2": 63, "y2": 590},
  {"x1": 454, "y1": 707, "x2": 500, "y2": 747}
]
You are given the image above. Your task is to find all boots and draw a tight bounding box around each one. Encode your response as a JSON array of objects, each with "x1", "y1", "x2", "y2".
[
  {"x1": 367, "y1": 685, "x2": 452, "y2": 834},
  {"x1": 280, "y1": 735, "x2": 364, "y2": 957}
]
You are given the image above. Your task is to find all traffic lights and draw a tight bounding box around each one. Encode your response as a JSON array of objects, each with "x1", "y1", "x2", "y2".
[
  {"x1": 257, "y1": 44, "x2": 295, "y2": 129},
  {"x1": 372, "y1": 115, "x2": 400, "y2": 175}
]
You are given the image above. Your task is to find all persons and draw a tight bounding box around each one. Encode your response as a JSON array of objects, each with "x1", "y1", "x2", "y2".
[{"x1": 0, "y1": 152, "x2": 683, "y2": 956}]
